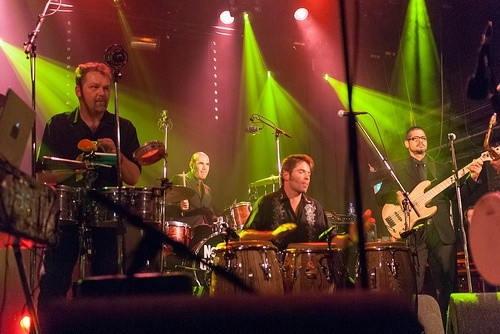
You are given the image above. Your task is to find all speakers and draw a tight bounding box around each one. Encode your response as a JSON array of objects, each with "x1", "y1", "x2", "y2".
[{"x1": 38, "y1": 298, "x2": 416, "y2": 334}]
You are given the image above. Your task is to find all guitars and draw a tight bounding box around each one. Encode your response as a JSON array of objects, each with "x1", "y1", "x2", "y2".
[{"x1": 381, "y1": 145, "x2": 500, "y2": 238}]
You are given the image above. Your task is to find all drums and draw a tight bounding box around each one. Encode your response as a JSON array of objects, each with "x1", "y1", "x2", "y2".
[
  {"x1": 44, "y1": 183, "x2": 82, "y2": 229},
  {"x1": 347, "y1": 242, "x2": 418, "y2": 313},
  {"x1": 284, "y1": 240, "x2": 346, "y2": 295},
  {"x1": 208, "y1": 238, "x2": 284, "y2": 303},
  {"x1": 82, "y1": 183, "x2": 161, "y2": 229},
  {"x1": 163, "y1": 220, "x2": 191, "y2": 255},
  {"x1": 223, "y1": 201, "x2": 253, "y2": 231}
]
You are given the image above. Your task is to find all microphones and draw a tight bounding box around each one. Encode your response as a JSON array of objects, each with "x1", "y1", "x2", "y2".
[
  {"x1": 245, "y1": 126, "x2": 259, "y2": 133},
  {"x1": 448, "y1": 133, "x2": 456, "y2": 142},
  {"x1": 338, "y1": 110, "x2": 368, "y2": 117},
  {"x1": 158, "y1": 110, "x2": 173, "y2": 132},
  {"x1": 104, "y1": 44, "x2": 128, "y2": 72}
]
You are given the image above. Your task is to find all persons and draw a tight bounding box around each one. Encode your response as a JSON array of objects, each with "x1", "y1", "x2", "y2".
[
  {"x1": 166, "y1": 151, "x2": 219, "y2": 274},
  {"x1": 236, "y1": 153, "x2": 371, "y2": 265},
  {"x1": 35, "y1": 61, "x2": 142, "y2": 333},
  {"x1": 377, "y1": 126, "x2": 482, "y2": 334}
]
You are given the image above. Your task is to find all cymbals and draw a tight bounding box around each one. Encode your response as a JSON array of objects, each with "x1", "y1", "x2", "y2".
[
  {"x1": 159, "y1": 183, "x2": 196, "y2": 204},
  {"x1": 191, "y1": 224, "x2": 233, "y2": 287},
  {"x1": 249, "y1": 173, "x2": 278, "y2": 189}
]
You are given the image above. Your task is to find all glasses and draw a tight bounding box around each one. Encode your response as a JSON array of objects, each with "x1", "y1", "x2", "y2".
[{"x1": 407, "y1": 136, "x2": 428, "y2": 142}]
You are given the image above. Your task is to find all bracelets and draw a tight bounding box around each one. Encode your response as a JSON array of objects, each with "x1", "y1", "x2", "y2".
[
  {"x1": 118, "y1": 153, "x2": 125, "y2": 165},
  {"x1": 347, "y1": 234, "x2": 357, "y2": 245}
]
